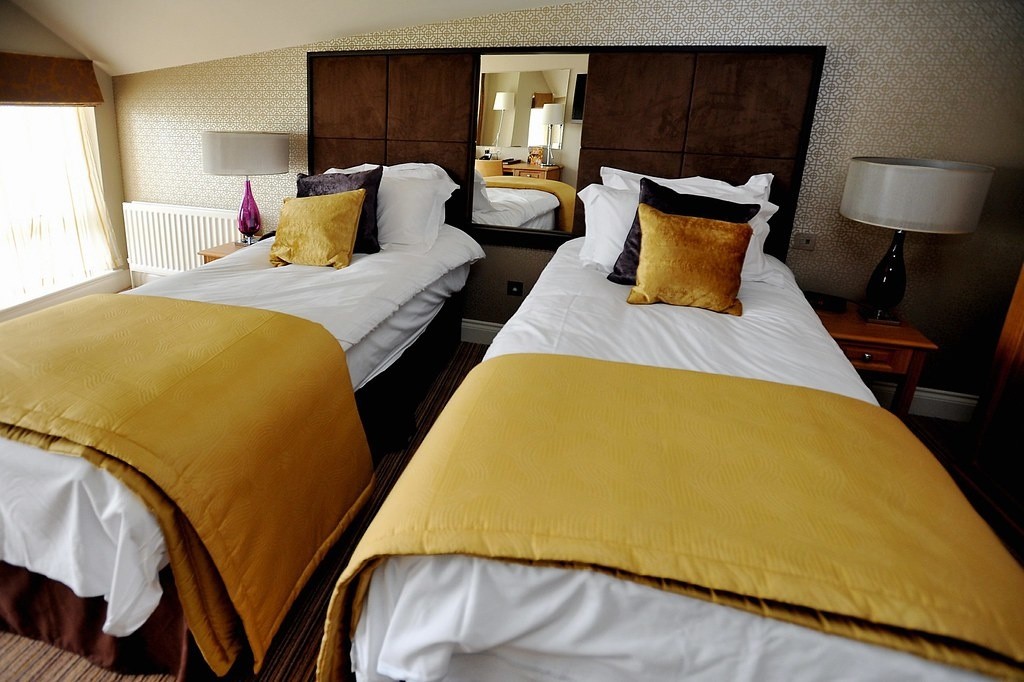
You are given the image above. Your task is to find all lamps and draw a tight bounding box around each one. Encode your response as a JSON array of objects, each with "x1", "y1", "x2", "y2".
[
  {"x1": 839, "y1": 157, "x2": 995, "y2": 326},
  {"x1": 201, "y1": 130, "x2": 290, "y2": 246},
  {"x1": 539, "y1": 103, "x2": 564, "y2": 166},
  {"x1": 492, "y1": 92, "x2": 515, "y2": 159}
]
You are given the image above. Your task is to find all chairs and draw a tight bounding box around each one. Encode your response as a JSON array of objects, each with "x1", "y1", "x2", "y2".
[{"x1": 475, "y1": 160, "x2": 503, "y2": 178}]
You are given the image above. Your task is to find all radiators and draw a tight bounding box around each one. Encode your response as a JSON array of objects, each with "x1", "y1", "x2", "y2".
[{"x1": 122, "y1": 201, "x2": 242, "y2": 277}]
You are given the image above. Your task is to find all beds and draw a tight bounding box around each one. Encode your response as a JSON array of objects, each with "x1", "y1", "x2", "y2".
[
  {"x1": 0, "y1": 218, "x2": 487, "y2": 682},
  {"x1": 472, "y1": 169, "x2": 576, "y2": 232},
  {"x1": 314, "y1": 235, "x2": 1024, "y2": 682}
]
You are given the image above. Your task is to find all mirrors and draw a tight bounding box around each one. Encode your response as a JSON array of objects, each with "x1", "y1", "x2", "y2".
[
  {"x1": 476, "y1": 69, "x2": 570, "y2": 150},
  {"x1": 471, "y1": 53, "x2": 589, "y2": 232}
]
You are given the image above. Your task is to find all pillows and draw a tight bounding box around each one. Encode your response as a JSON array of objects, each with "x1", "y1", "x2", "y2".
[
  {"x1": 323, "y1": 162, "x2": 460, "y2": 226},
  {"x1": 374, "y1": 175, "x2": 449, "y2": 254},
  {"x1": 269, "y1": 188, "x2": 367, "y2": 270},
  {"x1": 600, "y1": 166, "x2": 774, "y2": 201},
  {"x1": 625, "y1": 204, "x2": 753, "y2": 317},
  {"x1": 607, "y1": 176, "x2": 761, "y2": 287},
  {"x1": 297, "y1": 163, "x2": 383, "y2": 254},
  {"x1": 576, "y1": 183, "x2": 781, "y2": 286}
]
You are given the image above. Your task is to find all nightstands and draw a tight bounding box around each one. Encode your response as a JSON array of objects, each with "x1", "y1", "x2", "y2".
[
  {"x1": 198, "y1": 241, "x2": 247, "y2": 264},
  {"x1": 802, "y1": 289, "x2": 940, "y2": 425}
]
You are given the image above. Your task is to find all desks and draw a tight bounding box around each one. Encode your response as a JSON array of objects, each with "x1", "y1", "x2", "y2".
[{"x1": 503, "y1": 162, "x2": 564, "y2": 181}]
